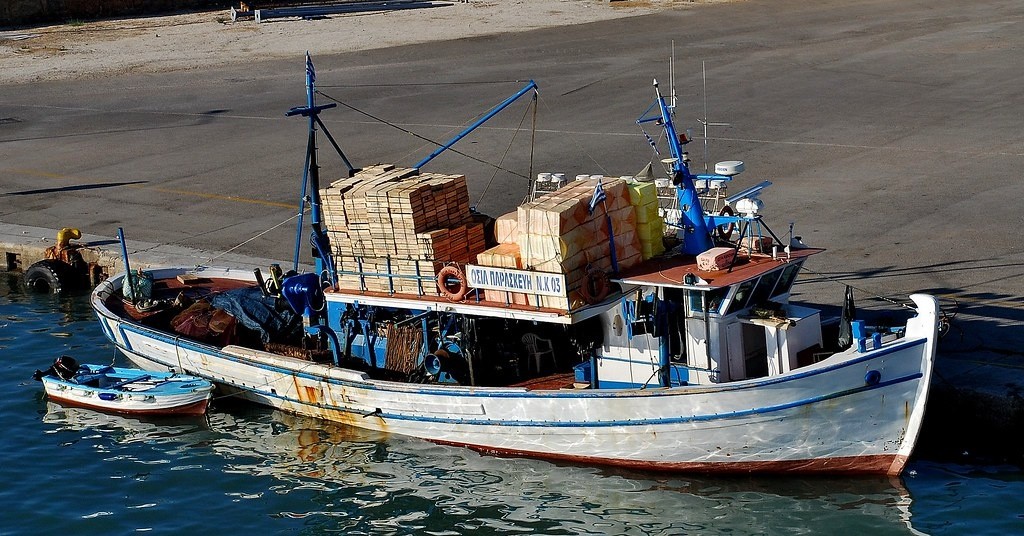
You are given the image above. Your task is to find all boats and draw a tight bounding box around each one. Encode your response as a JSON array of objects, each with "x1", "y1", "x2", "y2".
[
  {"x1": 32, "y1": 355, "x2": 217, "y2": 418},
  {"x1": 89, "y1": 51, "x2": 951, "y2": 482}
]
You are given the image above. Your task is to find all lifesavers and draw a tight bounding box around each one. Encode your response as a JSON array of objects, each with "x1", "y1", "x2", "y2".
[
  {"x1": 438, "y1": 265, "x2": 467, "y2": 301},
  {"x1": 23, "y1": 260, "x2": 72, "y2": 294}
]
